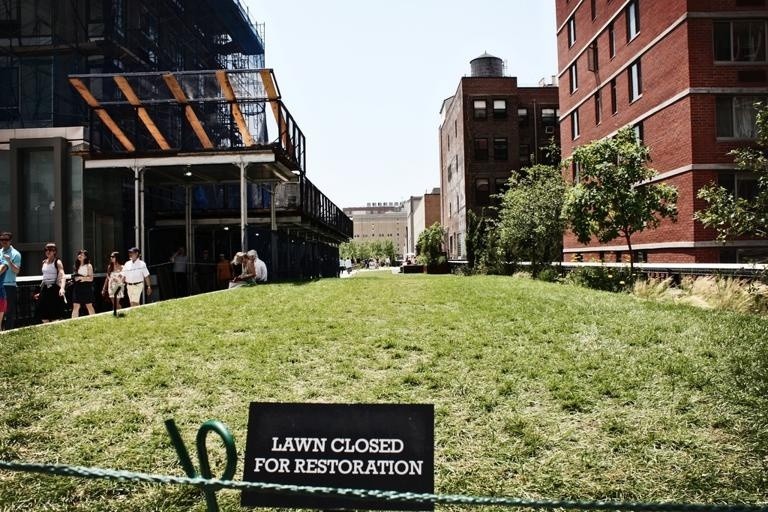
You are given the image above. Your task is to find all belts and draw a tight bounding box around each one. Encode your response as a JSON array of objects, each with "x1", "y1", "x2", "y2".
[{"x1": 126, "y1": 281, "x2": 142, "y2": 285}]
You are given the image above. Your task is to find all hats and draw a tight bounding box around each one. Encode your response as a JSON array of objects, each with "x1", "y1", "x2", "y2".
[{"x1": 128, "y1": 247, "x2": 139, "y2": 253}]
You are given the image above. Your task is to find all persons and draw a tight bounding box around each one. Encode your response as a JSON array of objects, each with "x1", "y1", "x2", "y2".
[
  {"x1": 217, "y1": 252, "x2": 234, "y2": 290},
  {"x1": 407, "y1": 256, "x2": 412, "y2": 265},
  {"x1": 67, "y1": 248, "x2": 96, "y2": 318},
  {"x1": 198, "y1": 249, "x2": 213, "y2": 293},
  {"x1": 0, "y1": 231, "x2": 22, "y2": 330},
  {"x1": 340, "y1": 256, "x2": 398, "y2": 273},
  {"x1": 412, "y1": 256, "x2": 418, "y2": 265},
  {"x1": 0, "y1": 259, "x2": 8, "y2": 331},
  {"x1": 168, "y1": 246, "x2": 187, "y2": 297},
  {"x1": 100, "y1": 251, "x2": 124, "y2": 309},
  {"x1": 35, "y1": 243, "x2": 66, "y2": 324},
  {"x1": 231, "y1": 252, "x2": 256, "y2": 283},
  {"x1": 120, "y1": 247, "x2": 152, "y2": 306},
  {"x1": 248, "y1": 249, "x2": 268, "y2": 284}
]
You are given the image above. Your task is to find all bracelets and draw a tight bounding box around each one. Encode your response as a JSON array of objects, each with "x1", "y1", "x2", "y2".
[
  {"x1": 10, "y1": 263, "x2": 13, "y2": 265},
  {"x1": 147, "y1": 286, "x2": 151, "y2": 289}
]
